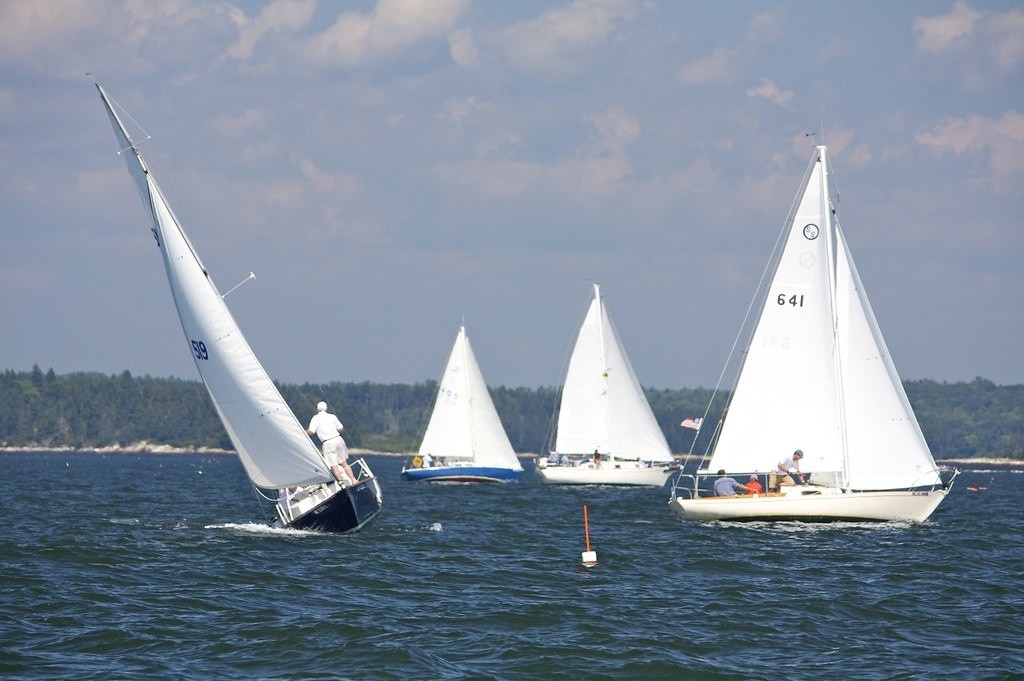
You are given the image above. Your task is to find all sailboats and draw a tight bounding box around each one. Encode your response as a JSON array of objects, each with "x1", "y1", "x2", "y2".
[
  {"x1": 531, "y1": 285, "x2": 687, "y2": 488},
  {"x1": 399, "y1": 316, "x2": 525, "y2": 485},
  {"x1": 664, "y1": 101, "x2": 962, "y2": 524},
  {"x1": 83, "y1": 69, "x2": 385, "y2": 536}
]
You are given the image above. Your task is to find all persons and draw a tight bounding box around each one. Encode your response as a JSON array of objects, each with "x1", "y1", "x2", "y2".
[
  {"x1": 592, "y1": 449, "x2": 604, "y2": 470},
  {"x1": 433, "y1": 456, "x2": 444, "y2": 468},
  {"x1": 305, "y1": 401, "x2": 359, "y2": 489},
  {"x1": 713, "y1": 469, "x2": 758, "y2": 497},
  {"x1": 745, "y1": 474, "x2": 762, "y2": 495},
  {"x1": 772, "y1": 449, "x2": 809, "y2": 493},
  {"x1": 421, "y1": 452, "x2": 433, "y2": 468}
]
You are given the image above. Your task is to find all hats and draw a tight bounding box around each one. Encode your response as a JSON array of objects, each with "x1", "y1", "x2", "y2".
[
  {"x1": 795, "y1": 450, "x2": 804, "y2": 459},
  {"x1": 750, "y1": 475, "x2": 758, "y2": 482},
  {"x1": 317, "y1": 401, "x2": 327, "y2": 413}
]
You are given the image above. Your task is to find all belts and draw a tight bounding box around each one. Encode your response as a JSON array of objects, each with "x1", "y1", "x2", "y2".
[{"x1": 322, "y1": 435, "x2": 341, "y2": 443}]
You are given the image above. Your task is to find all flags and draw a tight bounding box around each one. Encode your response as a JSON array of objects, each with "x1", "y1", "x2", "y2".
[{"x1": 680, "y1": 415, "x2": 703, "y2": 431}]
